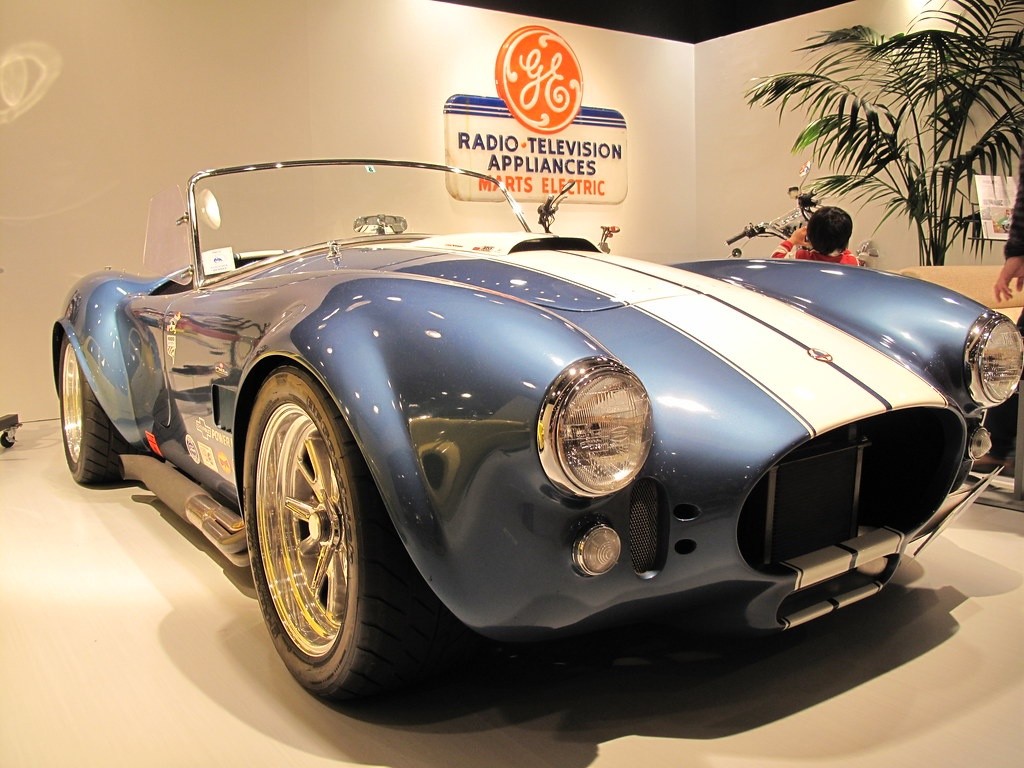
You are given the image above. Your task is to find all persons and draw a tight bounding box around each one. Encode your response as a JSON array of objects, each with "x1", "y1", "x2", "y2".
[
  {"x1": 769, "y1": 207, "x2": 857, "y2": 265},
  {"x1": 972, "y1": 150, "x2": 1024, "y2": 473}
]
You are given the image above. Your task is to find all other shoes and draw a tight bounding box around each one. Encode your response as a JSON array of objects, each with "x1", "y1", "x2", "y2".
[{"x1": 963, "y1": 455, "x2": 1008, "y2": 473}]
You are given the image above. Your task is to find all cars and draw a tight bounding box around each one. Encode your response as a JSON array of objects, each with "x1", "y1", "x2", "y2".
[{"x1": 51, "y1": 158, "x2": 1023, "y2": 710}]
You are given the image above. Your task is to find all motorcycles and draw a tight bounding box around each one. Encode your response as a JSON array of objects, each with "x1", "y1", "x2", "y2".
[{"x1": 725, "y1": 161, "x2": 878, "y2": 267}]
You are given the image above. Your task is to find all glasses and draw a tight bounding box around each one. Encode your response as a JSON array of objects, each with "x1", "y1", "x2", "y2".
[{"x1": 804, "y1": 235, "x2": 811, "y2": 242}]
